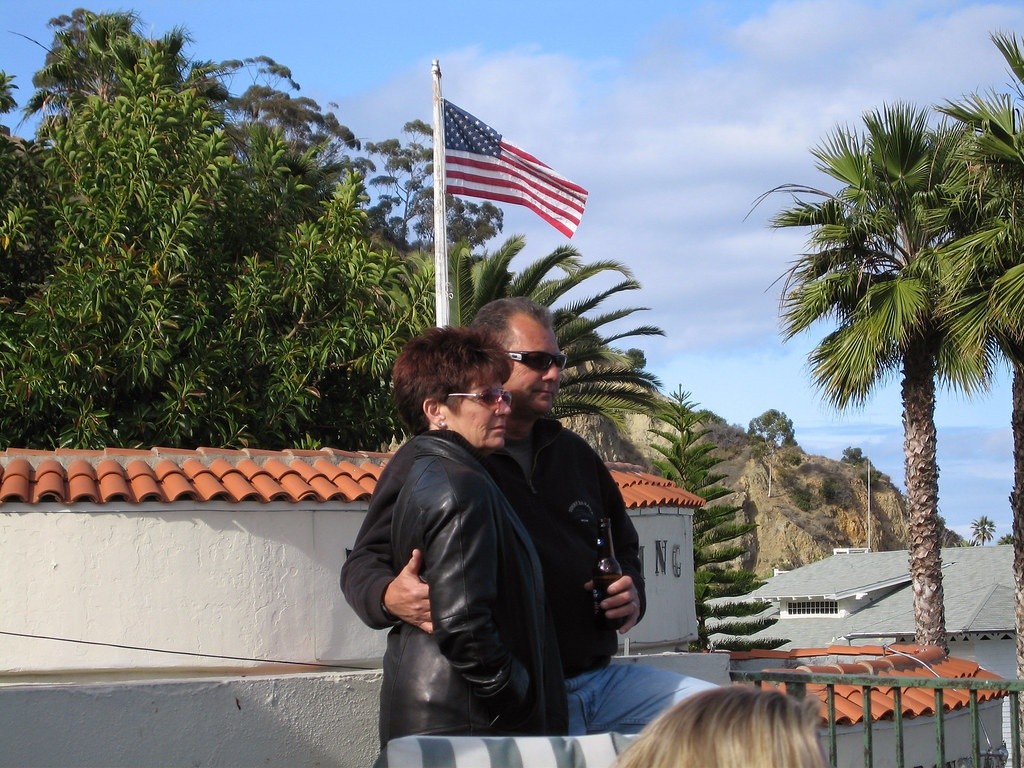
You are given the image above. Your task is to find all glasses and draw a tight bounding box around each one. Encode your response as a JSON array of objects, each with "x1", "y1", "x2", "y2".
[
  {"x1": 446, "y1": 387, "x2": 512, "y2": 408},
  {"x1": 501, "y1": 351, "x2": 568, "y2": 372}
]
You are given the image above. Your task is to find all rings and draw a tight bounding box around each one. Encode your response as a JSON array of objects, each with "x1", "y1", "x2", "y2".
[{"x1": 632, "y1": 601, "x2": 637, "y2": 612}]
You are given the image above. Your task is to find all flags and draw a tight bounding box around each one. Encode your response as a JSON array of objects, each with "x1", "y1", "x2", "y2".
[{"x1": 443, "y1": 100, "x2": 588, "y2": 240}]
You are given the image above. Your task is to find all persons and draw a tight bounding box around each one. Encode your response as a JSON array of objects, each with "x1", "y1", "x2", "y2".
[
  {"x1": 376, "y1": 325, "x2": 569, "y2": 739},
  {"x1": 339, "y1": 297, "x2": 723, "y2": 735},
  {"x1": 621, "y1": 684, "x2": 833, "y2": 768}
]
